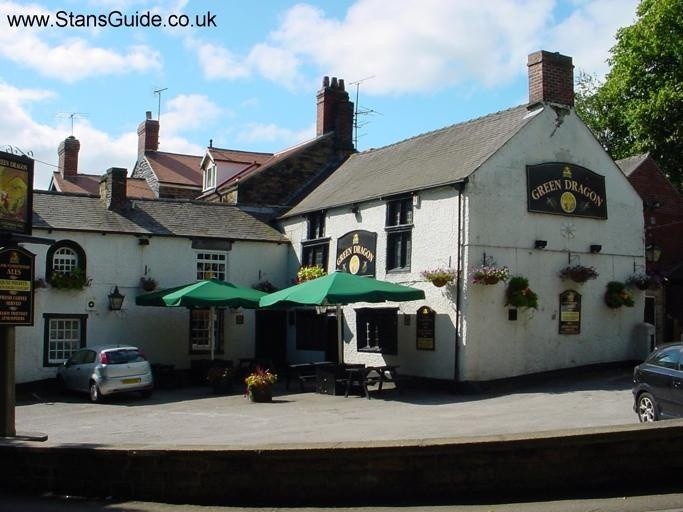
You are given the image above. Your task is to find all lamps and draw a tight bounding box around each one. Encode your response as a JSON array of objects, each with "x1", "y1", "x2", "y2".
[{"x1": 106, "y1": 284, "x2": 125, "y2": 311}]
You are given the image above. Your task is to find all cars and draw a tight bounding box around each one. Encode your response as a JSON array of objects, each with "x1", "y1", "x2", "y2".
[
  {"x1": 629, "y1": 339, "x2": 682, "y2": 425},
  {"x1": 55, "y1": 343, "x2": 154, "y2": 402}
]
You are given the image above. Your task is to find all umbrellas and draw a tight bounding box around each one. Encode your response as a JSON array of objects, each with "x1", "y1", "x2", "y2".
[
  {"x1": 257, "y1": 269, "x2": 425, "y2": 364},
  {"x1": 133, "y1": 276, "x2": 269, "y2": 360}
]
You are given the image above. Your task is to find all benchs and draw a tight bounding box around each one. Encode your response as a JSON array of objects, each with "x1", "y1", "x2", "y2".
[{"x1": 285, "y1": 360, "x2": 403, "y2": 400}]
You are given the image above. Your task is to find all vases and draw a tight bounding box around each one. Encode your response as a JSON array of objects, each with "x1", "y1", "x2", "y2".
[
  {"x1": 432, "y1": 278, "x2": 447, "y2": 288},
  {"x1": 634, "y1": 280, "x2": 649, "y2": 290},
  {"x1": 212, "y1": 383, "x2": 233, "y2": 395},
  {"x1": 484, "y1": 277, "x2": 497, "y2": 285},
  {"x1": 251, "y1": 388, "x2": 272, "y2": 403},
  {"x1": 570, "y1": 273, "x2": 586, "y2": 282}
]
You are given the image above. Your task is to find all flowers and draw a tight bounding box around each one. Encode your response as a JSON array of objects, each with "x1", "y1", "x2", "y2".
[
  {"x1": 206, "y1": 367, "x2": 234, "y2": 383},
  {"x1": 559, "y1": 264, "x2": 598, "y2": 280},
  {"x1": 421, "y1": 268, "x2": 455, "y2": 281},
  {"x1": 471, "y1": 265, "x2": 510, "y2": 285},
  {"x1": 242, "y1": 369, "x2": 277, "y2": 398},
  {"x1": 625, "y1": 268, "x2": 653, "y2": 284}
]
